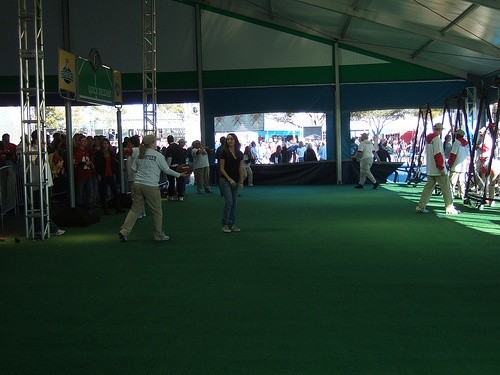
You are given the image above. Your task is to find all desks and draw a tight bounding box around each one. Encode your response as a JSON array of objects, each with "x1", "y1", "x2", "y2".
[{"x1": 210, "y1": 160, "x2": 404, "y2": 185}]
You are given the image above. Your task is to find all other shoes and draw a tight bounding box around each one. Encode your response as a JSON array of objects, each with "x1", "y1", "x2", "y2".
[
  {"x1": 205, "y1": 189, "x2": 213, "y2": 193},
  {"x1": 88, "y1": 210, "x2": 97, "y2": 215},
  {"x1": 179, "y1": 197, "x2": 184, "y2": 201},
  {"x1": 372, "y1": 182, "x2": 379, "y2": 189},
  {"x1": 167, "y1": 196, "x2": 175, "y2": 200},
  {"x1": 355, "y1": 184, "x2": 363, "y2": 188},
  {"x1": 248, "y1": 183, "x2": 254, "y2": 186}
]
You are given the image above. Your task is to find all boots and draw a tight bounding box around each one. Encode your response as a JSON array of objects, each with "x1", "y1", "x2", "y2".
[
  {"x1": 102, "y1": 202, "x2": 111, "y2": 214},
  {"x1": 115, "y1": 200, "x2": 125, "y2": 213}
]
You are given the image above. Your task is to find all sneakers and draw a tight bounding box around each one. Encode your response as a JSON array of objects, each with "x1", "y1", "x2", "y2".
[
  {"x1": 221, "y1": 226, "x2": 231, "y2": 232},
  {"x1": 153, "y1": 236, "x2": 169, "y2": 241},
  {"x1": 232, "y1": 227, "x2": 240, "y2": 232},
  {"x1": 119, "y1": 230, "x2": 127, "y2": 241},
  {"x1": 51, "y1": 229, "x2": 65, "y2": 235},
  {"x1": 416, "y1": 206, "x2": 429, "y2": 213},
  {"x1": 446, "y1": 209, "x2": 457, "y2": 214},
  {"x1": 35, "y1": 231, "x2": 47, "y2": 234}
]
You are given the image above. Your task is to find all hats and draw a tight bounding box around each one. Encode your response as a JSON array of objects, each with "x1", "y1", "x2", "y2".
[
  {"x1": 434, "y1": 124, "x2": 444, "y2": 129},
  {"x1": 178, "y1": 139, "x2": 187, "y2": 143},
  {"x1": 454, "y1": 129, "x2": 465, "y2": 136},
  {"x1": 143, "y1": 135, "x2": 161, "y2": 144}
]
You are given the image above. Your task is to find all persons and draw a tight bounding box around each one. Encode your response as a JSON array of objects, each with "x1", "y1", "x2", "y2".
[
  {"x1": 0, "y1": 130, "x2": 327, "y2": 240},
  {"x1": 351, "y1": 123, "x2": 500, "y2": 215}
]
